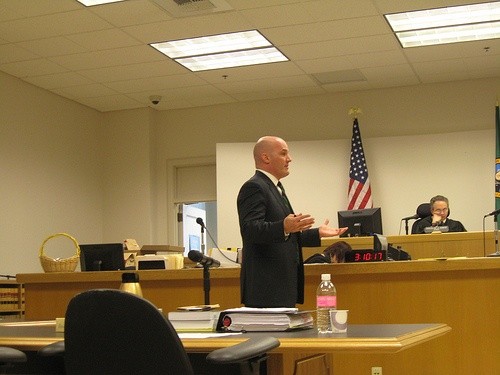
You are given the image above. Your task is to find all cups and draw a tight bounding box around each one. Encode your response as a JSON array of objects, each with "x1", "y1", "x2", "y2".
[{"x1": 329, "y1": 310, "x2": 349, "y2": 333}]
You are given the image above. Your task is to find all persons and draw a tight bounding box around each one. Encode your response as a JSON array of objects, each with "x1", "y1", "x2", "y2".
[
  {"x1": 236, "y1": 135, "x2": 348, "y2": 308},
  {"x1": 303, "y1": 241, "x2": 352, "y2": 264},
  {"x1": 415, "y1": 195, "x2": 467, "y2": 234}
]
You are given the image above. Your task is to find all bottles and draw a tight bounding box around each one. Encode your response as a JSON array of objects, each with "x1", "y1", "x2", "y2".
[{"x1": 316, "y1": 274, "x2": 337, "y2": 334}]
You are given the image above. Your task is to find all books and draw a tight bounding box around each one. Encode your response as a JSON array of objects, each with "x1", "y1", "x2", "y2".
[{"x1": 166, "y1": 309, "x2": 316, "y2": 333}]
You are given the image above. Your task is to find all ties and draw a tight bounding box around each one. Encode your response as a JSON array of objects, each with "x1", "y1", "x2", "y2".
[{"x1": 277, "y1": 181, "x2": 290, "y2": 213}]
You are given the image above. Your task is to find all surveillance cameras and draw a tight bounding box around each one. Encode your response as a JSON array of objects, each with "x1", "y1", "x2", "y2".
[{"x1": 149, "y1": 95, "x2": 161, "y2": 104}]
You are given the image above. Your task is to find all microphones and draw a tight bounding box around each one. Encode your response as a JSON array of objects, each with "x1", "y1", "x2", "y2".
[
  {"x1": 196, "y1": 217, "x2": 205, "y2": 228},
  {"x1": 485, "y1": 209, "x2": 500, "y2": 217},
  {"x1": 402, "y1": 215, "x2": 420, "y2": 220},
  {"x1": 188, "y1": 250, "x2": 220, "y2": 267}
]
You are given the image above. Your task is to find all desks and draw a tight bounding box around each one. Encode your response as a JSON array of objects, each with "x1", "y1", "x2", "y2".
[{"x1": 0, "y1": 231, "x2": 500, "y2": 375}]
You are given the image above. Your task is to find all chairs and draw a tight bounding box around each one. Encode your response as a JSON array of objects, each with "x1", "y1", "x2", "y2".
[{"x1": 38, "y1": 288, "x2": 280, "y2": 375}]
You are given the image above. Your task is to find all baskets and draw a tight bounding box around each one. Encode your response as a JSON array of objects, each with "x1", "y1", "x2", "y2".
[{"x1": 39, "y1": 232, "x2": 80, "y2": 272}]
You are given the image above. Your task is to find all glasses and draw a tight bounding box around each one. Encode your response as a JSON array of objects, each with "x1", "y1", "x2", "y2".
[{"x1": 432, "y1": 207, "x2": 448, "y2": 214}]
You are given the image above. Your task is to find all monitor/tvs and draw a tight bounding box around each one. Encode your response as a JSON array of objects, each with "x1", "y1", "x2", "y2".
[
  {"x1": 337, "y1": 207, "x2": 383, "y2": 238},
  {"x1": 79, "y1": 243, "x2": 126, "y2": 271}
]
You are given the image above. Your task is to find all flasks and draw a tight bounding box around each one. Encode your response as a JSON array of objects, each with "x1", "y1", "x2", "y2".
[{"x1": 119, "y1": 273, "x2": 143, "y2": 299}]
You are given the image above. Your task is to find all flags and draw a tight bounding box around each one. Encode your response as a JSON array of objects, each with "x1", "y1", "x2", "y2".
[{"x1": 346, "y1": 118, "x2": 374, "y2": 210}]
